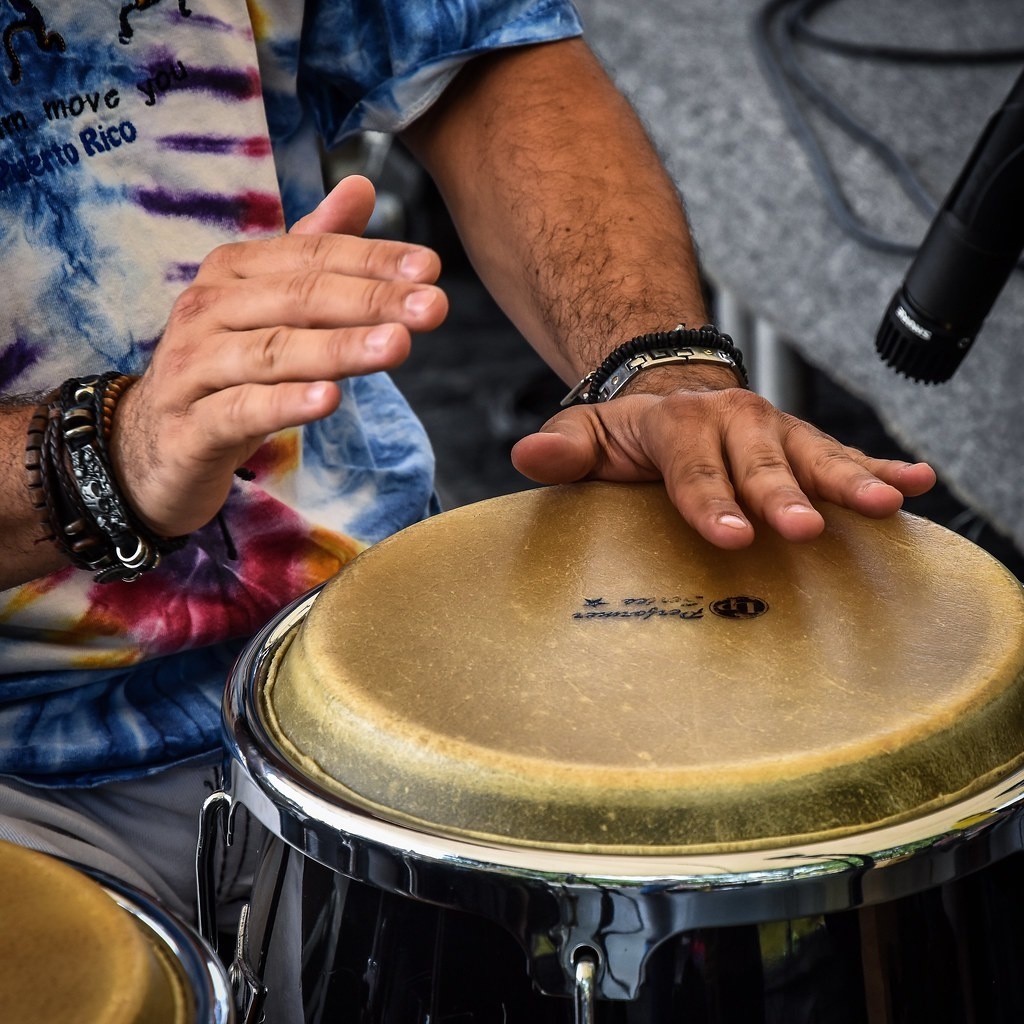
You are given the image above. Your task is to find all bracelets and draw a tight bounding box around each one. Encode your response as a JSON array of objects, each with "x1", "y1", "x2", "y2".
[
  {"x1": 562, "y1": 323, "x2": 752, "y2": 402},
  {"x1": 24, "y1": 372, "x2": 257, "y2": 587}
]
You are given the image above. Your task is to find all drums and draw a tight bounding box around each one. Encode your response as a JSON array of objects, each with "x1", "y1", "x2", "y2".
[
  {"x1": 201, "y1": 477, "x2": 1024, "y2": 1024},
  {"x1": 0, "y1": 834, "x2": 230, "y2": 1024}
]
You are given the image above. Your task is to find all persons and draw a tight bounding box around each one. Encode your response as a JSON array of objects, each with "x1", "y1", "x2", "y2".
[{"x1": 0, "y1": 0, "x2": 935, "y2": 1024}]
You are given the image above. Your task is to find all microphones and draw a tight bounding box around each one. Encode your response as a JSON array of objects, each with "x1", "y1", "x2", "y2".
[{"x1": 873, "y1": 67, "x2": 1024, "y2": 386}]
